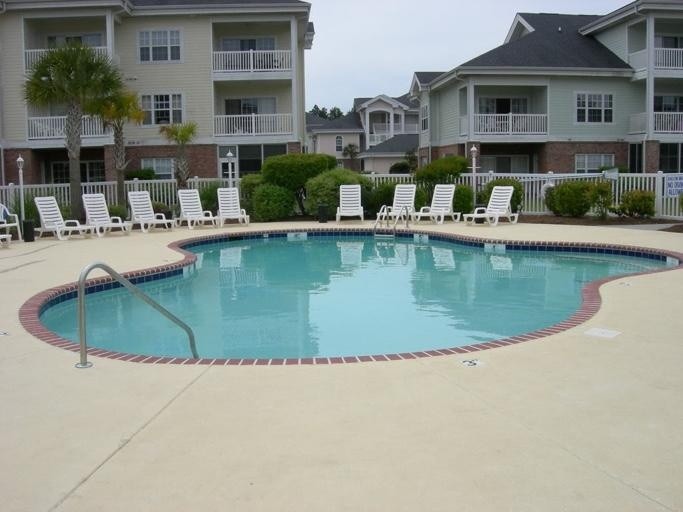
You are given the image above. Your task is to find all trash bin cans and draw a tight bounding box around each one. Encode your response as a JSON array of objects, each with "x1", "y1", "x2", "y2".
[
  {"x1": 23, "y1": 219, "x2": 35, "y2": 243},
  {"x1": 474, "y1": 204, "x2": 487, "y2": 224},
  {"x1": 161, "y1": 211, "x2": 172, "y2": 229},
  {"x1": 318, "y1": 204, "x2": 330, "y2": 223}
]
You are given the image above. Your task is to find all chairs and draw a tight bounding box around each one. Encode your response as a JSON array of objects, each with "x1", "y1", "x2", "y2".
[
  {"x1": 0, "y1": 183, "x2": 250, "y2": 244},
  {"x1": 181, "y1": 244, "x2": 249, "y2": 276},
  {"x1": 332, "y1": 179, "x2": 522, "y2": 229},
  {"x1": 336, "y1": 237, "x2": 515, "y2": 273}
]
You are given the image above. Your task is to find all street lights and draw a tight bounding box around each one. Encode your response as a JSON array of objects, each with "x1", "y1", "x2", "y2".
[
  {"x1": 226, "y1": 148, "x2": 236, "y2": 188},
  {"x1": 16, "y1": 153, "x2": 25, "y2": 238},
  {"x1": 466, "y1": 144, "x2": 484, "y2": 211}
]
